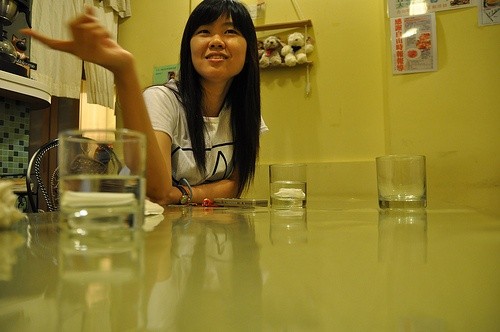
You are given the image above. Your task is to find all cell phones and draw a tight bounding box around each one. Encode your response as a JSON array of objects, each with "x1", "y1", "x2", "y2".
[{"x1": 213, "y1": 197, "x2": 268, "y2": 208}]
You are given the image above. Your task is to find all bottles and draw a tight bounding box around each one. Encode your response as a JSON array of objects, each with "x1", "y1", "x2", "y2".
[{"x1": 0, "y1": 37, "x2": 18, "y2": 63}]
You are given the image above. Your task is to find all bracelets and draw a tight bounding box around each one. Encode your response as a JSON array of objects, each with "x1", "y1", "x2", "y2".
[
  {"x1": 184, "y1": 178, "x2": 192, "y2": 199},
  {"x1": 177, "y1": 185, "x2": 192, "y2": 205},
  {"x1": 187, "y1": 206, "x2": 192, "y2": 224}
]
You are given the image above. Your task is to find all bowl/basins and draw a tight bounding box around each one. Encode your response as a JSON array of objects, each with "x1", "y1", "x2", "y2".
[{"x1": 0, "y1": 0, "x2": 20, "y2": 26}]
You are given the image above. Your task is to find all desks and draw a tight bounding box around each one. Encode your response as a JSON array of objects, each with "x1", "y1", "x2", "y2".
[{"x1": 0, "y1": 207, "x2": 500, "y2": 332}]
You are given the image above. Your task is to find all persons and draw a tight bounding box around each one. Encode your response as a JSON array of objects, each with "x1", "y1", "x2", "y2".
[
  {"x1": 19, "y1": 0, "x2": 269, "y2": 207},
  {"x1": 137, "y1": 205, "x2": 265, "y2": 332},
  {"x1": 169, "y1": 73, "x2": 175, "y2": 79}
]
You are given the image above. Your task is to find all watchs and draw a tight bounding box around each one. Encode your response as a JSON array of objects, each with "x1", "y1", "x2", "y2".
[
  {"x1": 179, "y1": 207, "x2": 187, "y2": 224},
  {"x1": 175, "y1": 186, "x2": 188, "y2": 205}
]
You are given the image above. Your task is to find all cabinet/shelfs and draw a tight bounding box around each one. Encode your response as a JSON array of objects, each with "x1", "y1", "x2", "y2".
[{"x1": 256, "y1": 19, "x2": 312, "y2": 66}]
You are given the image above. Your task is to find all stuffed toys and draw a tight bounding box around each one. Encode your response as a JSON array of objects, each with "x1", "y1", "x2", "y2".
[
  {"x1": 256, "y1": 39, "x2": 264, "y2": 59},
  {"x1": 259, "y1": 36, "x2": 287, "y2": 68},
  {"x1": 281, "y1": 33, "x2": 314, "y2": 67}
]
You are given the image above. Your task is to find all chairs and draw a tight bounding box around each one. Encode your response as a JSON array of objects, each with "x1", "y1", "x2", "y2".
[{"x1": 26, "y1": 137, "x2": 124, "y2": 214}]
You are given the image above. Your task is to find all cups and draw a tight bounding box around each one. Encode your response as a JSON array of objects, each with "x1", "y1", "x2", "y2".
[
  {"x1": 375, "y1": 154, "x2": 427, "y2": 208},
  {"x1": 270, "y1": 210, "x2": 309, "y2": 248},
  {"x1": 376, "y1": 208, "x2": 428, "y2": 277},
  {"x1": 268, "y1": 163, "x2": 308, "y2": 209},
  {"x1": 59, "y1": 253, "x2": 147, "y2": 332},
  {"x1": 57, "y1": 127, "x2": 148, "y2": 252}
]
